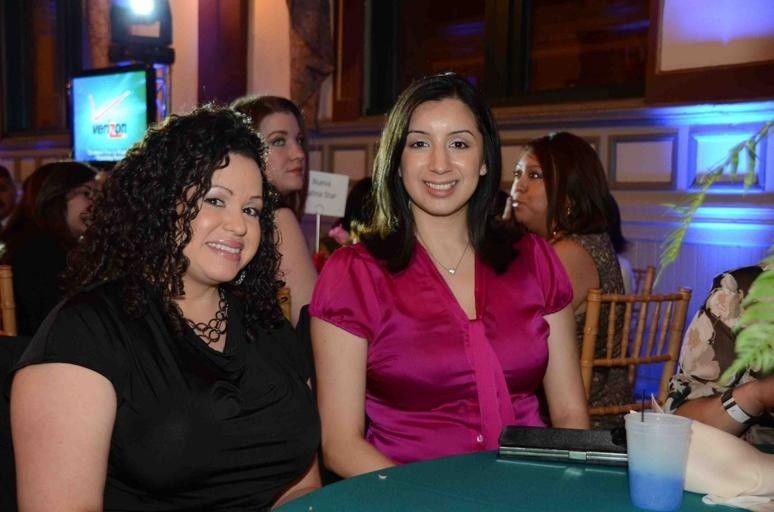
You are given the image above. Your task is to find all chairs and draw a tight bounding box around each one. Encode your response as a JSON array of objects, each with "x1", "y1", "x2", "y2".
[
  {"x1": 0, "y1": 264, "x2": 17, "y2": 337},
  {"x1": 626, "y1": 266, "x2": 656, "y2": 395},
  {"x1": 580, "y1": 286, "x2": 693, "y2": 419}
]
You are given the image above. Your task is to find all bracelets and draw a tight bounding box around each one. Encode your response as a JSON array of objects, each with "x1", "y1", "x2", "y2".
[{"x1": 721, "y1": 392, "x2": 763, "y2": 426}]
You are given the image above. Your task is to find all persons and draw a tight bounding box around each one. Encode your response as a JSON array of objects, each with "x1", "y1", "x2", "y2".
[
  {"x1": 0, "y1": 163, "x2": 100, "y2": 265},
  {"x1": 675, "y1": 377, "x2": 774, "y2": 436},
  {"x1": 0, "y1": 165, "x2": 15, "y2": 264},
  {"x1": 230, "y1": 95, "x2": 318, "y2": 329},
  {"x1": 496, "y1": 132, "x2": 628, "y2": 417},
  {"x1": 309, "y1": 72, "x2": 592, "y2": 479},
  {"x1": 4, "y1": 114, "x2": 321, "y2": 512}
]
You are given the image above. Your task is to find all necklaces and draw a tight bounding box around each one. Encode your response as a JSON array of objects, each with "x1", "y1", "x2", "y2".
[
  {"x1": 417, "y1": 233, "x2": 472, "y2": 275},
  {"x1": 186, "y1": 288, "x2": 231, "y2": 345}
]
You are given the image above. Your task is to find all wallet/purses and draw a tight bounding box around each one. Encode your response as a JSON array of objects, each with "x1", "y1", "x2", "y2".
[{"x1": 497, "y1": 424, "x2": 627, "y2": 467}]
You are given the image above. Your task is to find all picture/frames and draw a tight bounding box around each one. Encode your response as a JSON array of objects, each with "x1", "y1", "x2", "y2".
[
  {"x1": 607, "y1": 135, "x2": 677, "y2": 189},
  {"x1": 329, "y1": 145, "x2": 369, "y2": 182},
  {"x1": 500, "y1": 140, "x2": 526, "y2": 188},
  {"x1": 583, "y1": 138, "x2": 598, "y2": 155},
  {"x1": 688, "y1": 126, "x2": 767, "y2": 197},
  {"x1": 305, "y1": 146, "x2": 324, "y2": 172}
]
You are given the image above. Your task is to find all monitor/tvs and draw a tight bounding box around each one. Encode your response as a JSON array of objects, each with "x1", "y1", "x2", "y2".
[{"x1": 67, "y1": 62, "x2": 158, "y2": 171}]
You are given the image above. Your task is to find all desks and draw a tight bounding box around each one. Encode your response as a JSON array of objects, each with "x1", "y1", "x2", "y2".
[{"x1": 272, "y1": 440, "x2": 774, "y2": 512}]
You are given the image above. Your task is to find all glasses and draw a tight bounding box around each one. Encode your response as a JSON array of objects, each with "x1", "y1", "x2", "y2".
[{"x1": 63, "y1": 185, "x2": 104, "y2": 203}]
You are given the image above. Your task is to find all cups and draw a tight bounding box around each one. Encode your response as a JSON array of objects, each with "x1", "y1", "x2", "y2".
[{"x1": 623, "y1": 413, "x2": 692, "y2": 511}]
